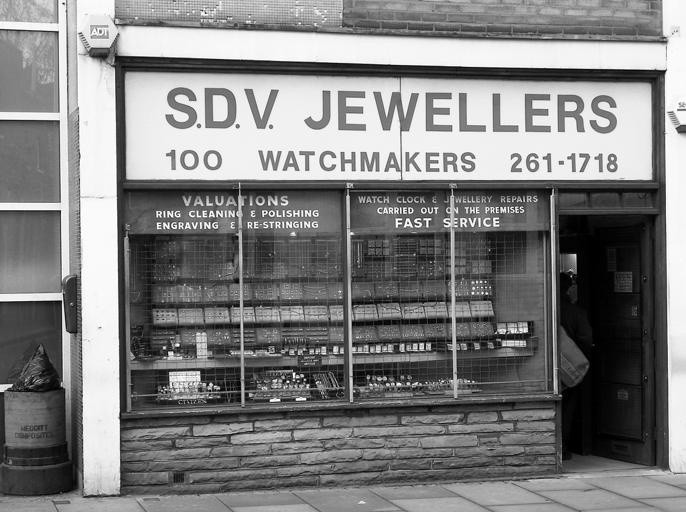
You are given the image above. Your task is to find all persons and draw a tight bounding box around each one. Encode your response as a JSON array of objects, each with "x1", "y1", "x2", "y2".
[{"x1": 563, "y1": 270, "x2": 595, "y2": 458}]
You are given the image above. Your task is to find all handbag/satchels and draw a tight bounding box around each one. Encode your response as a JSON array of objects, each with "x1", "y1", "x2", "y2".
[{"x1": 557, "y1": 326, "x2": 590, "y2": 388}]
